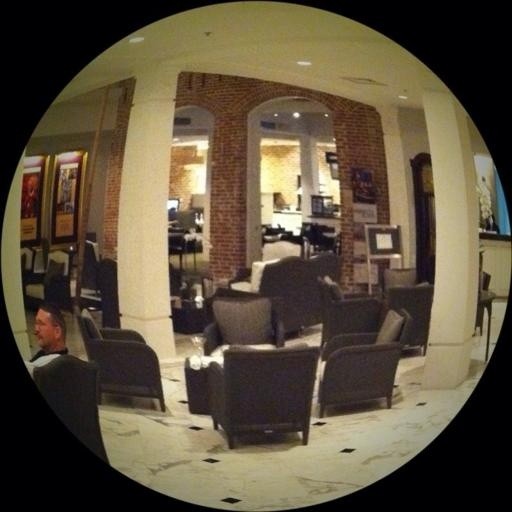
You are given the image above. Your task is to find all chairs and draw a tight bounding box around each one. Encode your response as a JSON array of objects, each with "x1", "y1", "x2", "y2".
[{"x1": 169, "y1": 211, "x2": 199, "y2": 272}]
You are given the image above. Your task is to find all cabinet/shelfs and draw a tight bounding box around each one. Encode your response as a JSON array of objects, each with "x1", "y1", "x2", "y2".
[{"x1": 409, "y1": 150, "x2": 438, "y2": 284}]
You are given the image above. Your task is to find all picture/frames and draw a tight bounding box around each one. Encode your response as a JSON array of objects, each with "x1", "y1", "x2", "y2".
[{"x1": 18, "y1": 149, "x2": 90, "y2": 247}]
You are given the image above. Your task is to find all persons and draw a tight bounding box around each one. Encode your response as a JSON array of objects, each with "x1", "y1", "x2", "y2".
[{"x1": 20, "y1": 304, "x2": 69, "y2": 381}]
